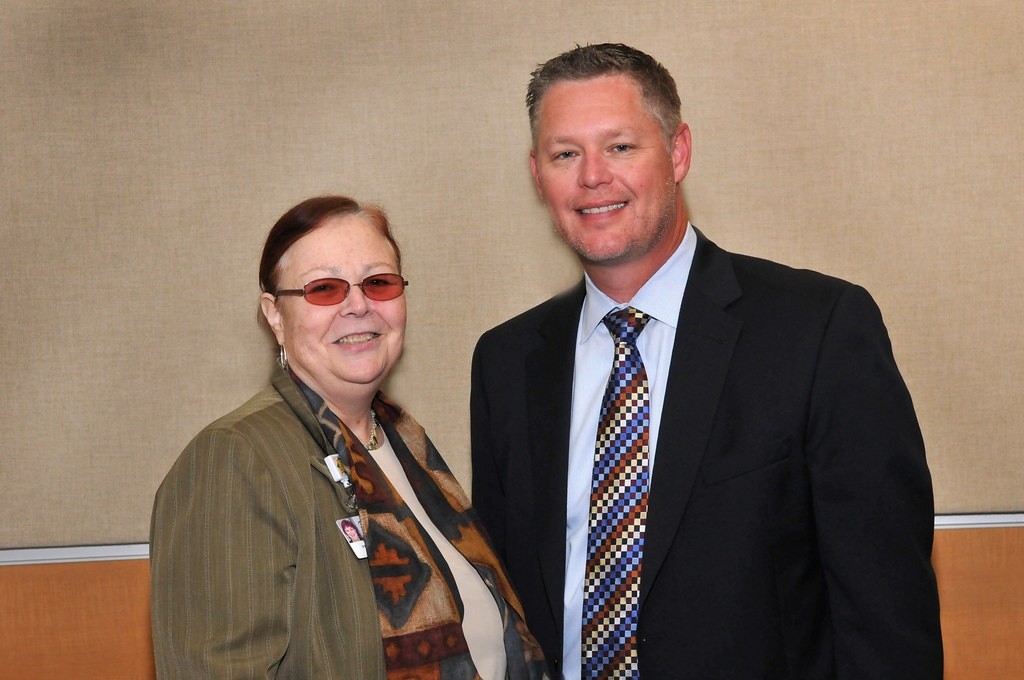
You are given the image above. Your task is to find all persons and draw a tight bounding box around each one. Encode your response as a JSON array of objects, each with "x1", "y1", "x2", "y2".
[
  {"x1": 469, "y1": 39, "x2": 949, "y2": 680},
  {"x1": 339, "y1": 519, "x2": 364, "y2": 543},
  {"x1": 149, "y1": 197, "x2": 552, "y2": 680}
]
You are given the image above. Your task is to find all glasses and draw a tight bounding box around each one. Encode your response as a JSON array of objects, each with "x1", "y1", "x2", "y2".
[{"x1": 276, "y1": 273, "x2": 408, "y2": 306}]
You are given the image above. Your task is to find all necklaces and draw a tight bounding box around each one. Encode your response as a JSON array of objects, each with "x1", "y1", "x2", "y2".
[{"x1": 361, "y1": 408, "x2": 380, "y2": 449}]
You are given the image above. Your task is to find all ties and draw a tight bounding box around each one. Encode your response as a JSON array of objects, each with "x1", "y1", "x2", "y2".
[{"x1": 580, "y1": 306, "x2": 652, "y2": 680}]
